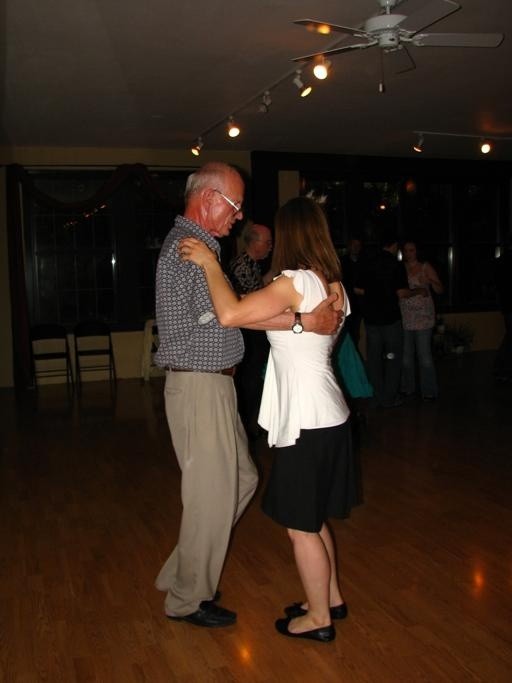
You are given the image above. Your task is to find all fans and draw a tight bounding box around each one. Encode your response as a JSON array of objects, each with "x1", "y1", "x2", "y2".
[{"x1": 291, "y1": 0, "x2": 506, "y2": 69}]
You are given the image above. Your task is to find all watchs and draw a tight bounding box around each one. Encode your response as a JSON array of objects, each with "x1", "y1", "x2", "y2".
[{"x1": 290, "y1": 311, "x2": 304, "y2": 334}]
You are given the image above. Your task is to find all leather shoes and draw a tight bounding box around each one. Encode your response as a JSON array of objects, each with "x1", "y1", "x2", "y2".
[
  {"x1": 164, "y1": 601, "x2": 238, "y2": 627},
  {"x1": 275, "y1": 618, "x2": 336, "y2": 642},
  {"x1": 284, "y1": 602, "x2": 347, "y2": 620}
]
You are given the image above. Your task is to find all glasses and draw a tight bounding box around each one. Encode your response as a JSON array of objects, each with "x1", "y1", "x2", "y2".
[
  {"x1": 217, "y1": 191, "x2": 245, "y2": 214},
  {"x1": 257, "y1": 239, "x2": 273, "y2": 246}
]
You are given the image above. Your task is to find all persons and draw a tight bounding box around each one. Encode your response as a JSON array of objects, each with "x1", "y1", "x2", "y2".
[
  {"x1": 361, "y1": 232, "x2": 430, "y2": 409},
  {"x1": 177, "y1": 192, "x2": 364, "y2": 641},
  {"x1": 395, "y1": 235, "x2": 445, "y2": 404},
  {"x1": 150, "y1": 157, "x2": 349, "y2": 623},
  {"x1": 223, "y1": 222, "x2": 272, "y2": 297},
  {"x1": 333, "y1": 330, "x2": 378, "y2": 415}
]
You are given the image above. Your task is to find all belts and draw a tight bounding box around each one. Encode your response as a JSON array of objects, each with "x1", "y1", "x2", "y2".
[{"x1": 164, "y1": 364, "x2": 236, "y2": 379}]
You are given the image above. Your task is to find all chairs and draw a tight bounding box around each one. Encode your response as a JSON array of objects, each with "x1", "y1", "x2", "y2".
[
  {"x1": 69, "y1": 331, "x2": 119, "y2": 400},
  {"x1": 25, "y1": 331, "x2": 75, "y2": 410}
]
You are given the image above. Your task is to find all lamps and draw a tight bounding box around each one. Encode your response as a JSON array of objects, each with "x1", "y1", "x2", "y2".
[
  {"x1": 184, "y1": 58, "x2": 338, "y2": 162},
  {"x1": 410, "y1": 127, "x2": 491, "y2": 161}
]
